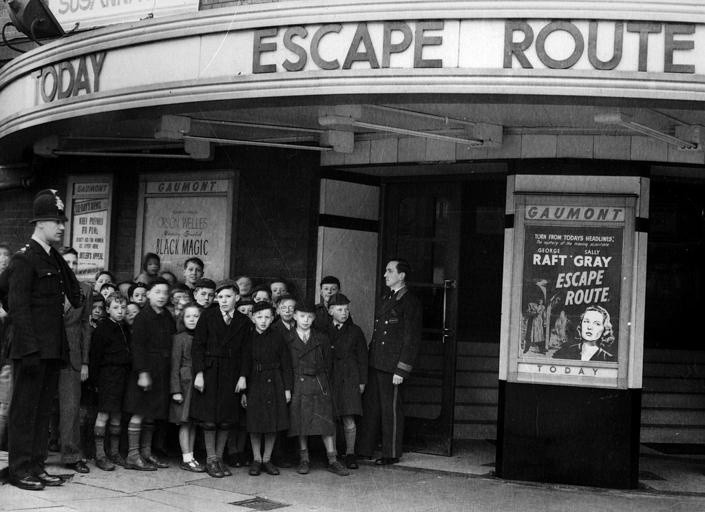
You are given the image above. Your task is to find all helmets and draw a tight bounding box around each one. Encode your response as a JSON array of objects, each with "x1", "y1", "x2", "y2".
[{"x1": 27, "y1": 187, "x2": 69, "y2": 224}]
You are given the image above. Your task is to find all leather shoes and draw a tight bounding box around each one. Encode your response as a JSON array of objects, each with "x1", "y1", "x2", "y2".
[
  {"x1": 95, "y1": 455, "x2": 116, "y2": 473},
  {"x1": 249, "y1": 461, "x2": 262, "y2": 475},
  {"x1": 205, "y1": 460, "x2": 223, "y2": 479},
  {"x1": 38, "y1": 470, "x2": 64, "y2": 486},
  {"x1": 218, "y1": 461, "x2": 232, "y2": 476},
  {"x1": 374, "y1": 456, "x2": 401, "y2": 465},
  {"x1": 346, "y1": 453, "x2": 359, "y2": 469},
  {"x1": 110, "y1": 453, "x2": 126, "y2": 467},
  {"x1": 262, "y1": 461, "x2": 281, "y2": 476},
  {"x1": 180, "y1": 457, "x2": 205, "y2": 473},
  {"x1": 66, "y1": 461, "x2": 90, "y2": 473},
  {"x1": 5, "y1": 470, "x2": 45, "y2": 490},
  {"x1": 145, "y1": 454, "x2": 168, "y2": 468},
  {"x1": 124, "y1": 454, "x2": 157, "y2": 471}
]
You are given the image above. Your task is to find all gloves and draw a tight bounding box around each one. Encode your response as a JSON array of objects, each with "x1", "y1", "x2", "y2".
[{"x1": 19, "y1": 354, "x2": 42, "y2": 375}]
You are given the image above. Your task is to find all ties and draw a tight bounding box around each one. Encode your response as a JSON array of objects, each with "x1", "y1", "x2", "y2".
[
  {"x1": 388, "y1": 290, "x2": 395, "y2": 300},
  {"x1": 302, "y1": 333, "x2": 308, "y2": 345},
  {"x1": 335, "y1": 324, "x2": 340, "y2": 331}
]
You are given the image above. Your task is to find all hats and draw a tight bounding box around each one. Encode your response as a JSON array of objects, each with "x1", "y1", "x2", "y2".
[
  {"x1": 252, "y1": 301, "x2": 272, "y2": 315},
  {"x1": 91, "y1": 270, "x2": 113, "y2": 308},
  {"x1": 194, "y1": 278, "x2": 216, "y2": 290},
  {"x1": 168, "y1": 282, "x2": 190, "y2": 298},
  {"x1": 295, "y1": 299, "x2": 316, "y2": 313},
  {"x1": 128, "y1": 282, "x2": 149, "y2": 295},
  {"x1": 248, "y1": 284, "x2": 272, "y2": 296},
  {"x1": 215, "y1": 278, "x2": 239, "y2": 292},
  {"x1": 321, "y1": 273, "x2": 352, "y2": 305},
  {"x1": 57, "y1": 246, "x2": 78, "y2": 257}
]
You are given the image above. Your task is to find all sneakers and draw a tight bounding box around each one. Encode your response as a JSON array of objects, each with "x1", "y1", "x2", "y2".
[
  {"x1": 326, "y1": 459, "x2": 350, "y2": 476},
  {"x1": 297, "y1": 461, "x2": 311, "y2": 474}
]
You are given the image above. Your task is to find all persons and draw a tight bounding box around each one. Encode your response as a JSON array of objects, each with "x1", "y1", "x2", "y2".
[
  {"x1": 553, "y1": 304, "x2": 616, "y2": 362},
  {"x1": 525, "y1": 295, "x2": 548, "y2": 353},
  {"x1": 548, "y1": 310, "x2": 569, "y2": 350},
  {"x1": 7, "y1": 187, "x2": 85, "y2": 489},
  {"x1": 368, "y1": 259, "x2": 422, "y2": 465}
]
